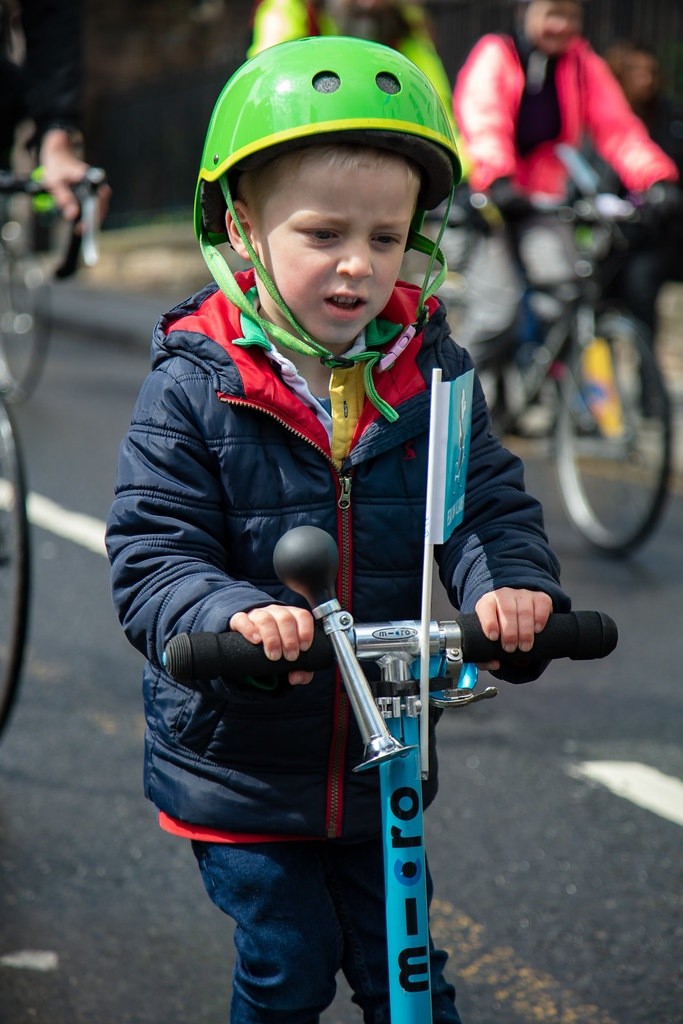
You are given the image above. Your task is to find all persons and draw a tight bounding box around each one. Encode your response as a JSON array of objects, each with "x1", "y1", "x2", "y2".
[
  {"x1": 454, "y1": 0, "x2": 683, "y2": 407},
  {"x1": 0, "y1": 0, "x2": 111, "y2": 236},
  {"x1": 107, "y1": 37, "x2": 571, "y2": 1023},
  {"x1": 250, "y1": 1, "x2": 473, "y2": 225}
]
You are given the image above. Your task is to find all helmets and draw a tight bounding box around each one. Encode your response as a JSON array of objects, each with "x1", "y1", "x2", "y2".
[{"x1": 193, "y1": 37, "x2": 466, "y2": 267}]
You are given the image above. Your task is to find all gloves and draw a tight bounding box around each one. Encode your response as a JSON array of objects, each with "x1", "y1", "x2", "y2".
[
  {"x1": 473, "y1": 176, "x2": 533, "y2": 239},
  {"x1": 641, "y1": 181, "x2": 683, "y2": 225}
]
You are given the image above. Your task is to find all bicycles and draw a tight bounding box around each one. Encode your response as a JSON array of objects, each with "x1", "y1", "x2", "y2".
[
  {"x1": 415, "y1": 189, "x2": 678, "y2": 556},
  {"x1": 0, "y1": 165, "x2": 109, "y2": 738}
]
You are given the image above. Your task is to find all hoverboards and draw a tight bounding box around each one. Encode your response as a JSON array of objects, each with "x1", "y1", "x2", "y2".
[{"x1": 157, "y1": 606, "x2": 618, "y2": 1024}]
